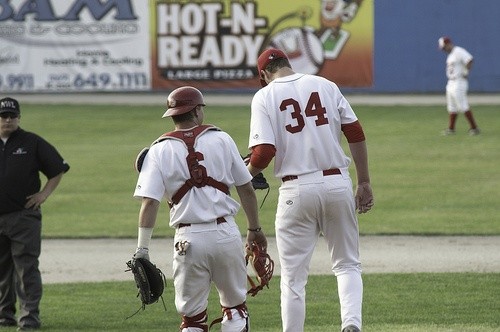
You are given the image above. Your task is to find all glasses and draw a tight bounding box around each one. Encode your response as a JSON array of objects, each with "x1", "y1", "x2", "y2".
[{"x1": 0, "y1": 113, "x2": 18, "y2": 119}]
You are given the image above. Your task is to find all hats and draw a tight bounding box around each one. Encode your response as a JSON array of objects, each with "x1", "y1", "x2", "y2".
[
  {"x1": 258, "y1": 49, "x2": 290, "y2": 87},
  {"x1": 0, "y1": 97, "x2": 19, "y2": 113},
  {"x1": 438, "y1": 37, "x2": 450, "y2": 49}
]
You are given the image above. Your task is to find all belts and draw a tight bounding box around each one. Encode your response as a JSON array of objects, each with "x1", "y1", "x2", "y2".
[
  {"x1": 282, "y1": 169, "x2": 341, "y2": 182},
  {"x1": 179, "y1": 217, "x2": 226, "y2": 229}
]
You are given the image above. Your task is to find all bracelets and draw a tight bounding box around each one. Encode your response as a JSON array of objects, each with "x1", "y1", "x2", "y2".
[{"x1": 248, "y1": 228, "x2": 262, "y2": 231}]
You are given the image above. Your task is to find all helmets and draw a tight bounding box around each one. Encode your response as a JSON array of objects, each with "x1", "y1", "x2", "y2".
[{"x1": 161, "y1": 86, "x2": 206, "y2": 118}]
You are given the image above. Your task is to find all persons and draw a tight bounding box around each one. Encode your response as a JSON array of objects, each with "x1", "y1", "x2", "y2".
[
  {"x1": 242, "y1": 49, "x2": 374, "y2": 332},
  {"x1": 0, "y1": 97, "x2": 70, "y2": 329},
  {"x1": 438, "y1": 36, "x2": 480, "y2": 137},
  {"x1": 130, "y1": 86, "x2": 269, "y2": 332}
]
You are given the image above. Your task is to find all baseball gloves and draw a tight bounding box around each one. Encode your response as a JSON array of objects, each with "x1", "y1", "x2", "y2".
[
  {"x1": 129, "y1": 257, "x2": 164, "y2": 304},
  {"x1": 242, "y1": 155, "x2": 270, "y2": 190}
]
